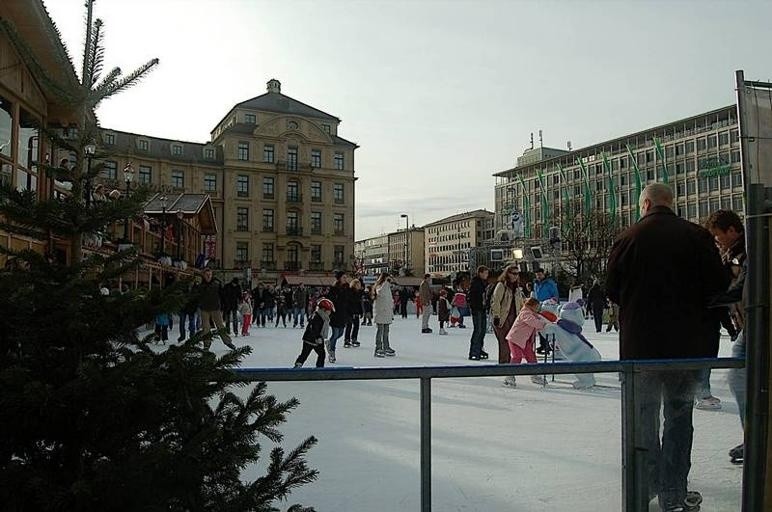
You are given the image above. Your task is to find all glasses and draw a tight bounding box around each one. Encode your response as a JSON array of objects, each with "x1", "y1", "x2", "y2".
[{"x1": 507, "y1": 270, "x2": 520, "y2": 276}]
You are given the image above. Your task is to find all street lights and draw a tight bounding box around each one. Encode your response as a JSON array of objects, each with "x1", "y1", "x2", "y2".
[
  {"x1": 159, "y1": 191, "x2": 170, "y2": 257},
  {"x1": 123, "y1": 159, "x2": 136, "y2": 242},
  {"x1": 362, "y1": 250, "x2": 367, "y2": 265},
  {"x1": 175, "y1": 206, "x2": 184, "y2": 262},
  {"x1": 84, "y1": 135, "x2": 95, "y2": 210},
  {"x1": 400, "y1": 213, "x2": 410, "y2": 270}
]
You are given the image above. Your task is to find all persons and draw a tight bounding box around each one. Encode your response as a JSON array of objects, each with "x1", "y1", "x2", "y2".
[
  {"x1": 438, "y1": 266, "x2": 618, "y2": 386},
  {"x1": 694, "y1": 369, "x2": 721, "y2": 410},
  {"x1": 57, "y1": 159, "x2": 173, "y2": 258},
  {"x1": 154, "y1": 268, "x2": 432, "y2": 369},
  {"x1": 604, "y1": 182, "x2": 727, "y2": 511},
  {"x1": 706, "y1": 209, "x2": 747, "y2": 460}
]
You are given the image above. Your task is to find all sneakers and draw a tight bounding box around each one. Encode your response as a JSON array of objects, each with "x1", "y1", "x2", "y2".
[
  {"x1": 727, "y1": 442, "x2": 745, "y2": 457},
  {"x1": 536, "y1": 345, "x2": 553, "y2": 352},
  {"x1": 698, "y1": 395, "x2": 721, "y2": 404},
  {"x1": 420, "y1": 323, "x2": 468, "y2": 335},
  {"x1": 504, "y1": 375, "x2": 516, "y2": 382},
  {"x1": 362, "y1": 322, "x2": 372, "y2": 324},
  {"x1": 374, "y1": 347, "x2": 395, "y2": 353},
  {"x1": 531, "y1": 375, "x2": 546, "y2": 383},
  {"x1": 469, "y1": 347, "x2": 488, "y2": 357},
  {"x1": 647, "y1": 490, "x2": 704, "y2": 512},
  {"x1": 152, "y1": 319, "x2": 305, "y2": 349},
  {"x1": 344, "y1": 338, "x2": 361, "y2": 345},
  {"x1": 325, "y1": 339, "x2": 338, "y2": 360}
]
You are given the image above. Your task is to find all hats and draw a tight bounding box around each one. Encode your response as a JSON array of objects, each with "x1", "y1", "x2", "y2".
[
  {"x1": 334, "y1": 270, "x2": 346, "y2": 280},
  {"x1": 439, "y1": 289, "x2": 449, "y2": 296}
]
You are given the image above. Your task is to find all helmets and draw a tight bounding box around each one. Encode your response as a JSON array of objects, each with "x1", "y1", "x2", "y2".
[{"x1": 318, "y1": 298, "x2": 336, "y2": 313}]
